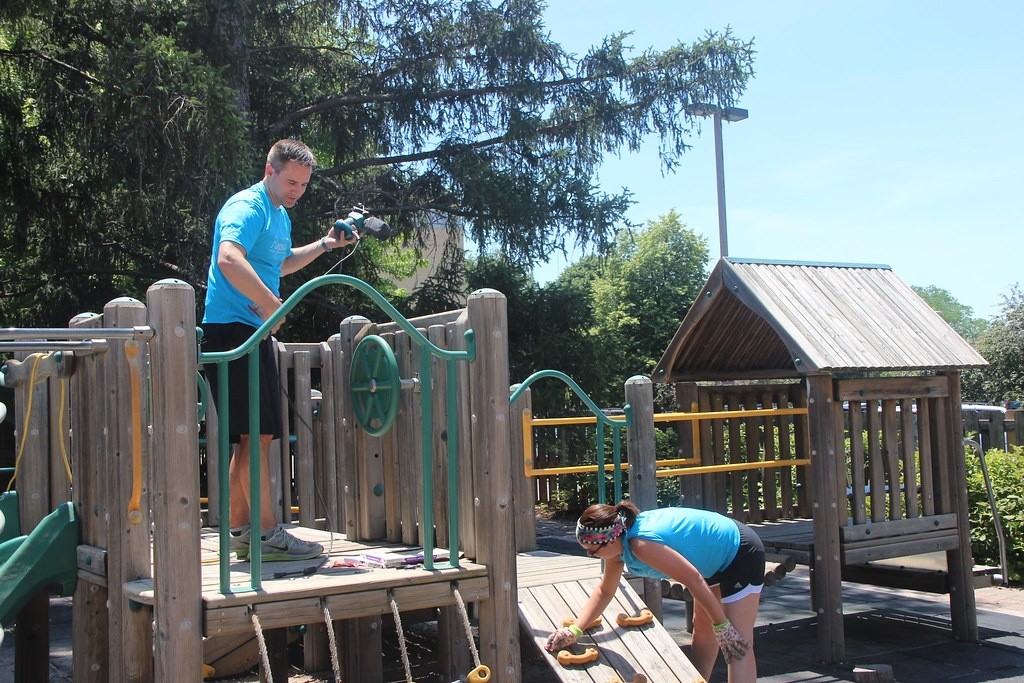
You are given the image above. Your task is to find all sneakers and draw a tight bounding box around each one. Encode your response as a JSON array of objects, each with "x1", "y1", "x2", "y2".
[
  {"x1": 247, "y1": 525, "x2": 325, "y2": 562},
  {"x1": 217, "y1": 526, "x2": 250, "y2": 557}
]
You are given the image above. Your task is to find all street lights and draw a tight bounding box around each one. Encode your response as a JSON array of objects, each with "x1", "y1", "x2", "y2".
[{"x1": 684, "y1": 102, "x2": 748, "y2": 257}]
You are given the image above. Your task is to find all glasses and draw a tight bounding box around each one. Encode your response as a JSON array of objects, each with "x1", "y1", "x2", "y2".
[{"x1": 586, "y1": 540, "x2": 608, "y2": 558}]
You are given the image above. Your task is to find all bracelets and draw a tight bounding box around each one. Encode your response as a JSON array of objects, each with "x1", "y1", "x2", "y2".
[{"x1": 321, "y1": 237, "x2": 332, "y2": 253}]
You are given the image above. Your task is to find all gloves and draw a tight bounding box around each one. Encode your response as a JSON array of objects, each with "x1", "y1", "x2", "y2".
[
  {"x1": 544, "y1": 624, "x2": 584, "y2": 652},
  {"x1": 711, "y1": 619, "x2": 752, "y2": 665}
]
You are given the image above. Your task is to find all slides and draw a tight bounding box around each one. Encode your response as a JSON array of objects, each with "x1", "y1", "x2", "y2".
[{"x1": 0, "y1": 502, "x2": 78, "y2": 618}]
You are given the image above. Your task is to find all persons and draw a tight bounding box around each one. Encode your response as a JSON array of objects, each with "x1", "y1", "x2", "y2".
[
  {"x1": 201, "y1": 139, "x2": 363, "y2": 561},
  {"x1": 545, "y1": 501, "x2": 766, "y2": 683}
]
